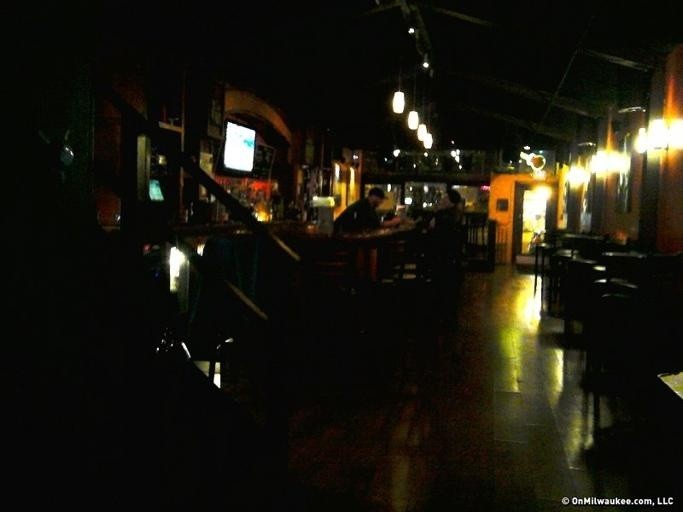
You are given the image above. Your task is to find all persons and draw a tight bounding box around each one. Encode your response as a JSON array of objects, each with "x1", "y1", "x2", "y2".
[
  {"x1": 333, "y1": 187, "x2": 401, "y2": 233},
  {"x1": 409, "y1": 189, "x2": 462, "y2": 271}
]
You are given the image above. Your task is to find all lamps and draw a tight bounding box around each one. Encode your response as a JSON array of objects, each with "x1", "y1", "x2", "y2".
[{"x1": 392, "y1": 0, "x2": 434, "y2": 149}]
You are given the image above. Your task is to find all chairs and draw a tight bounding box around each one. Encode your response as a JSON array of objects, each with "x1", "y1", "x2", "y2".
[
  {"x1": 307, "y1": 240, "x2": 352, "y2": 297},
  {"x1": 534, "y1": 230, "x2": 682, "y2": 418},
  {"x1": 371, "y1": 239, "x2": 433, "y2": 297}
]
